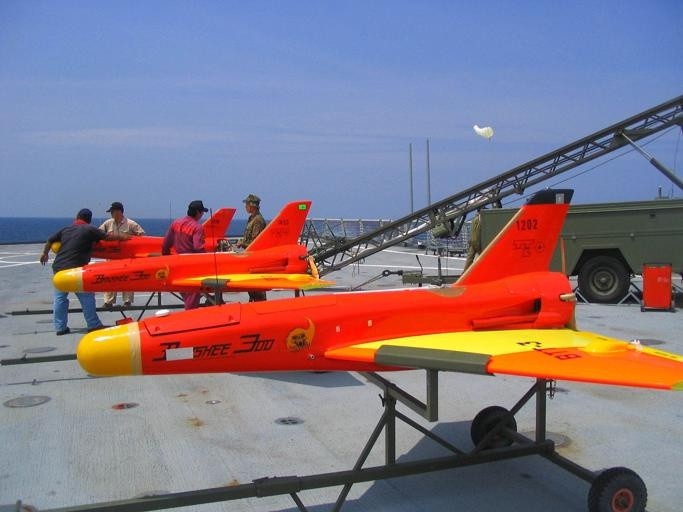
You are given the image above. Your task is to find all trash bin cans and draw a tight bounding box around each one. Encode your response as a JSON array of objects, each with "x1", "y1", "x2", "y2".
[{"x1": 641, "y1": 263, "x2": 675, "y2": 312}]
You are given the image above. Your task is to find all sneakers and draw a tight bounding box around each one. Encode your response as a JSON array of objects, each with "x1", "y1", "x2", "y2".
[
  {"x1": 57, "y1": 327, "x2": 69, "y2": 336},
  {"x1": 123, "y1": 302, "x2": 130, "y2": 307},
  {"x1": 102, "y1": 303, "x2": 112, "y2": 307}
]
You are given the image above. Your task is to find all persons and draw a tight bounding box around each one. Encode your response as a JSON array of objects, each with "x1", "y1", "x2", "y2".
[
  {"x1": 161, "y1": 200, "x2": 208, "y2": 310},
  {"x1": 99, "y1": 202, "x2": 145, "y2": 307},
  {"x1": 236, "y1": 194, "x2": 266, "y2": 302},
  {"x1": 40, "y1": 208, "x2": 133, "y2": 335}
]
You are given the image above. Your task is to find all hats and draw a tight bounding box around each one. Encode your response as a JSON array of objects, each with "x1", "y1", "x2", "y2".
[
  {"x1": 243, "y1": 195, "x2": 261, "y2": 209},
  {"x1": 106, "y1": 202, "x2": 122, "y2": 212},
  {"x1": 189, "y1": 201, "x2": 208, "y2": 211}
]
